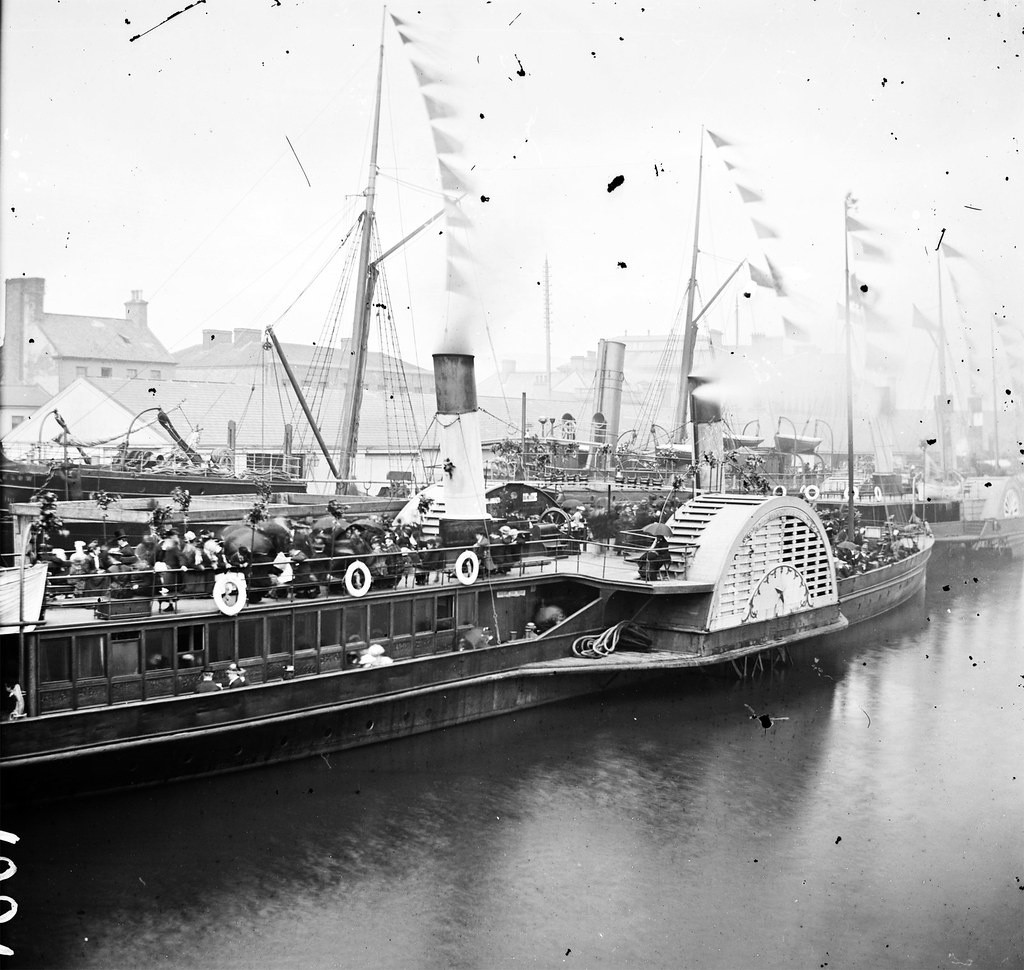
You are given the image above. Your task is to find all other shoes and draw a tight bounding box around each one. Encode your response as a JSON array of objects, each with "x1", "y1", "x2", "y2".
[{"x1": 164, "y1": 605, "x2": 173, "y2": 613}]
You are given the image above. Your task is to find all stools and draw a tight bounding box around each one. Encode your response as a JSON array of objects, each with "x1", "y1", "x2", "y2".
[{"x1": 658, "y1": 561, "x2": 673, "y2": 581}]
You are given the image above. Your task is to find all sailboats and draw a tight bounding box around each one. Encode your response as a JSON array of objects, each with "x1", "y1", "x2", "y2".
[{"x1": 0, "y1": 2, "x2": 1024, "y2": 810}]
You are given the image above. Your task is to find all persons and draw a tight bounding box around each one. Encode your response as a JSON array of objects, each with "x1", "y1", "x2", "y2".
[{"x1": 46, "y1": 497, "x2": 917, "y2": 695}]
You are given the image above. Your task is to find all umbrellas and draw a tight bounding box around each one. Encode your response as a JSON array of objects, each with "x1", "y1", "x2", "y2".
[
  {"x1": 535, "y1": 604, "x2": 561, "y2": 623},
  {"x1": 837, "y1": 541, "x2": 857, "y2": 549},
  {"x1": 233, "y1": 533, "x2": 272, "y2": 552},
  {"x1": 561, "y1": 499, "x2": 583, "y2": 508},
  {"x1": 314, "y1": 517, "x2": 350, "y2": 532},
  {"x1": 642, "y1": 522, "x2": 673, "y2": 537},
  {"x1": 259, "y1": 520, "x2": 290, "y2": 536},
  {"x1": 222, "y1": 524, "x2": 246, "y2": 536},
  {"x1": 345, "y1": 519, "x2": 386, "y2": 535},
  {"x1": 595, "y1": 497, "x2": 613, "y2": 506},
  {"x1": 226, "y1": 527, "x2": 254, "y2": 542}
]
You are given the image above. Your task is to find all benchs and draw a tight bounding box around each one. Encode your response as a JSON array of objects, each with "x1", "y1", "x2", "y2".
[
  {"x1": 97, "y1": 596, "x2": 154, "y2": 622},
  {"x1": 245, "y1": 558, "x2": 551, "y2": 605},
  {"x1": 639, "y1": 569, "x2": 685, "y2": 581},
  {"x1": 44, "y1": 581, "x2": 177, "y2": 613}
]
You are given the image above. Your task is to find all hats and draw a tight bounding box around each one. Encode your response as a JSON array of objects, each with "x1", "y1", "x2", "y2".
[
  {"x1": 370, "y1": 535, "x2": 382, "y2": 544},
  {"x1": 184, "y1": 531, "x2": 196, "y2": 542},
  {"x1": 576, "y1": 505, "x2": 586, "y2": 511},
  {"x1": 474, "y1": 526, "x2": 486, "y2": 534},
  {"x1": 385, "y1": 535, "x2": 398, "y2": 541},
  {"x1": 114, "y1": 529, "x2": 129, "y2": 542}
]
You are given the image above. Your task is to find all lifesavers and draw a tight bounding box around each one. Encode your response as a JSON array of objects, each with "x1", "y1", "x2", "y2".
[
  {"x1": 804, "y1": 484, "x2": 820, "y2": 500},
  {"x1": 800, "y1": 485, "x2": 807, "y2": 493},
  {"x1": 455, "y1": 551, "x2": 479, "y2": 585},
  {"x1": 506, "y1": 422, "x2": 519, "y2": 435},
  {"x1": 345, "y1": 561, "x2": 371, "y2": 597},
  {"x1": 213, "y1": 575, "x2": 247, "y2": 616},
  {"x1": 564, "y1": 421, "x2": 576, "y2": 434},
  {"x1": 773, "y1": 485, "x2": 786, "y2": 496},
  {"x1": 844, "y1": 486, "x2": 858, "y2": 499},
  {"x1": 874, "y1": 486, "x2": 882, "y2": 501},
  {"x1": 572, "y1": 450, "x2": 577, "y2": 458}
]
